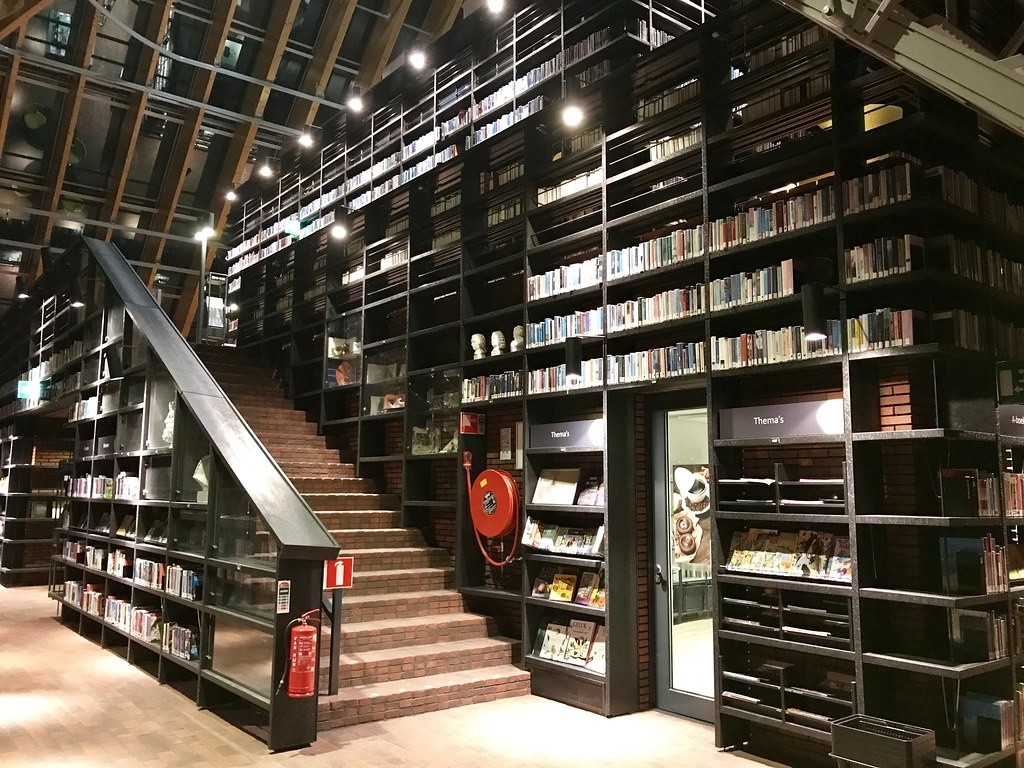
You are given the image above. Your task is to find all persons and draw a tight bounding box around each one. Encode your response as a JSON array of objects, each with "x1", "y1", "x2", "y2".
[
  {"x1": 509, "y1": 326, "x2": 524, "y2": 352},
  {"x1": 490, "y1": 331, "x2": 506, "y2": 357},
  {"x1": 161, "y1": 400, "x2": 175, "y2": 443},
  {"x1": 471, "y1": 333, "x2": 487, "y2": 360}
]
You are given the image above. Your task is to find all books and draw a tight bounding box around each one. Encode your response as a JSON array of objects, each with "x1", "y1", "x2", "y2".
[
  {"x1": 60, "y1": 528, "x2": 276, "y2": 601},
  {"x1": 223, "y1": 127, "x2": 526, "y2": 329},
  {"x1": 520, "y1": 515, "x2": 605, "y2": 558},
  {"x1": 0, "y1": 340, "x2": 118, "y2": 423},
  {"x1": 725, "y1": 528, "x2": 852, "y2": 584},
  {"x1": 844, "y1": 234, "x2": 1024, "y2": 298},
  {"x1": 530, "y1": 619, "x2": 607, "y2": 676},
  {"x1": 63, "y1": 580, "x2": 200, "y2": 661},
  {"x1": 533, "y1": 467, "x2": 605, "y2": 507},
  {"x1": 76, "y1": 511, "x2": 167, "y2": 545},
  {"x1": 936, "y1": 467, "x2": 1023, "y2": 753},
  {"x1": 736, "y1": 24, "x2": 833, "y2": 157},
  {"x1": 841, "y1": 166, "x2": 1024, "y2": 237},
  {"x1": 523, "y1": 186, "x2": 845, "y2": 393},
  {"x1": 51, "y1": 474, "x2": 139, "y2": 520},
  {"x1": 847, "y1": 308, "x2": 1024, "y2": 361},
  {"x1": 435, "y1": 16, "x2": 702, "y2": 226},
  {"x1": 530, "y1": 566, "x2": 605, "y2": 612}
]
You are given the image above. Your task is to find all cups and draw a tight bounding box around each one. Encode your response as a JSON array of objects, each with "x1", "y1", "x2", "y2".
[{"x1": 686, "y1": 474, "x2": 707, "y2": 503}]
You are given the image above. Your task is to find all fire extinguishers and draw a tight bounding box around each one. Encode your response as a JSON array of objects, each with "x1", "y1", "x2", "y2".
[{"x1": 276, "y1": 609, "x2": 320, "y2": 697}]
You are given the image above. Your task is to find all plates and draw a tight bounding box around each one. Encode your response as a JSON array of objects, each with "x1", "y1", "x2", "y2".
[{"x1": 681, "y1": 483, "x2": 710, "y2": 514}]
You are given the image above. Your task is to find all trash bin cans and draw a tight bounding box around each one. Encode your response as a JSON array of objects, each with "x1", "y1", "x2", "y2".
[{"x1": 828, "y1": 714, "x2": 937, "y2": 768}]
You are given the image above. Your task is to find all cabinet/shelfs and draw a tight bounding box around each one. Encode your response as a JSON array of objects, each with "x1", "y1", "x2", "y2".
[
  {"x1": 1, "y1": 238, "x2": 344, "y2": 751},
  {"x1": 220, "y1": 1, "x2": 1024, "y2": 768}
]
